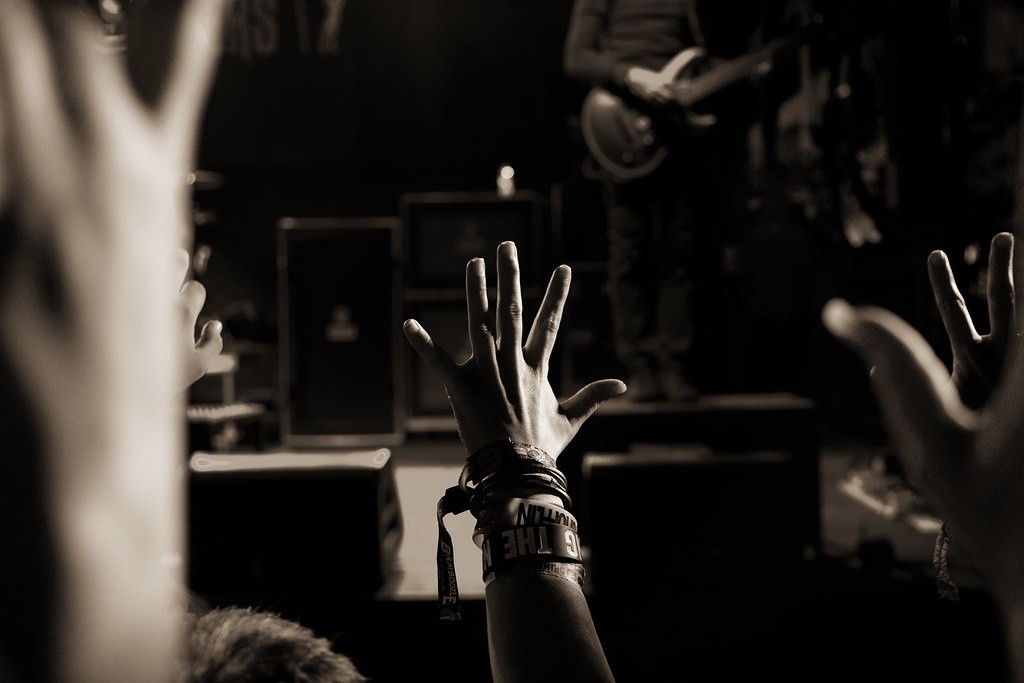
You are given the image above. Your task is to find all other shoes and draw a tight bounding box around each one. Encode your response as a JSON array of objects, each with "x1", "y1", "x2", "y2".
[
  {"x1": 654, "y1": 374, "x2": 698, "y2": 400},
  {"x1": 628, "y1": 360, "x2": 655, "y2": 400}
]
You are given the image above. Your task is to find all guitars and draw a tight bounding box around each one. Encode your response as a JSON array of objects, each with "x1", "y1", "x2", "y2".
[{"x1": 580, "y1": 33, "x2": 791, "y2": 182}]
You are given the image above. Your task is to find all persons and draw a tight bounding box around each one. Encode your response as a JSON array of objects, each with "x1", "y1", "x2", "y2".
[
  {"x1": 853, "y1": 234, "x2": 1024, "y2": 682},
  {"x1": 0, "y1": 0, "x2": 235, "y2": 682},
  {"x1": 189, "y1": 239, "x2": 628, "y2": 683},
  {"x1": 743, "y1": 0, "x2": 881, "y2": 401},
  {"x1": 561, "y1": 0, "x2": 714, "y2": 401}
]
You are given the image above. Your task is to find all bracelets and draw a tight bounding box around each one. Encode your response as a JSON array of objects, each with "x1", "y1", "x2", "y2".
[{"x1": 436, "y1": 437, "x2": 587, "y2": 622}]
